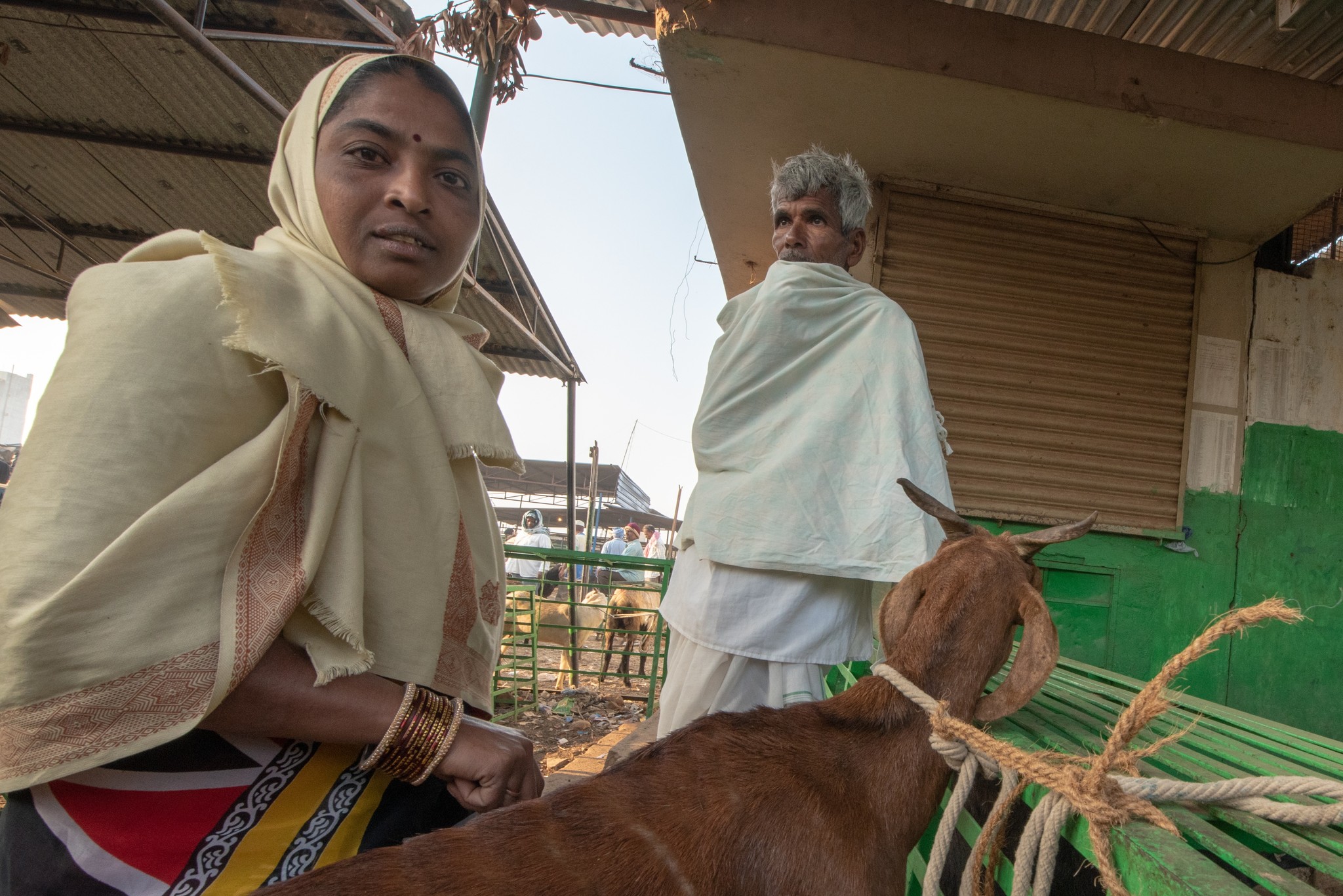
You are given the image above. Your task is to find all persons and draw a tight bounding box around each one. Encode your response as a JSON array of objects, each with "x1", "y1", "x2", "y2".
[
  {"x1": 596, "y1": 522, "x2": 666, "y2": 599},
  {"x1": 657, "y1": 141, "x2": 956, "y2": 742},
  {"x1": 504, "y1": 503, "x2": 551, "y2": 596},
  {"x1": 0, "y1": 52, "x2": 545, "y2": 896},
  {"x1": 554, "y1": 520, "x2": 587, "y2": 605}
]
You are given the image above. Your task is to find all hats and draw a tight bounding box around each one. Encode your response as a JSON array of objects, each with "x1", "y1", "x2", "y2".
[
  {"x1": 624, "y1": 523, "x2": 640, "y2": 539},
  {"x1": 504, "y1": 528, "x2": 514, "y2": 535},
  {"x1": 575, "y1": 520, "x2": 585, "y2": 528}
]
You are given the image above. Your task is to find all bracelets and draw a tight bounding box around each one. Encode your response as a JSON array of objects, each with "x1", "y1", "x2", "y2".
[{"x1": 355, "y1": 682, "x2": 462, "y2": 786}]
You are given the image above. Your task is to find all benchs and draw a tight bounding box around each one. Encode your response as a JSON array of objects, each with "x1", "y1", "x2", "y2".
[{"x1": 821, "y1": 640, "x2": 1343, "y2": 896}]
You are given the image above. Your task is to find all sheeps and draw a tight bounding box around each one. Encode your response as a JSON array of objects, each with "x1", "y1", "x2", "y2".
[
  {"x1": 493, "y1": 583, "x2": 609, "y2": 691},
  {"x1": 251, "y1": 477, "x2": 1101, "y2": 896},
  {"x1": 597, "y1": 583, "x2": 667, "y2": 689}
]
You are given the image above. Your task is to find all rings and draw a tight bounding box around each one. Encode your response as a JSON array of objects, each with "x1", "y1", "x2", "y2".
[{"x1": 505, "y1": 790, "x2": 522, "y2": 797}]
